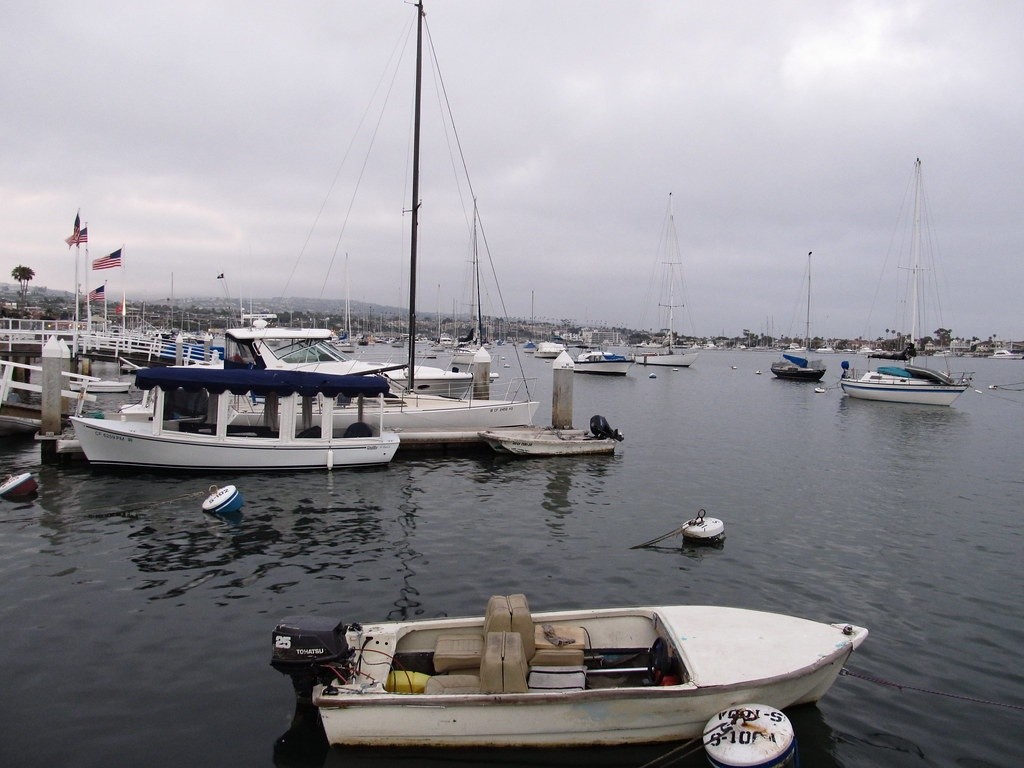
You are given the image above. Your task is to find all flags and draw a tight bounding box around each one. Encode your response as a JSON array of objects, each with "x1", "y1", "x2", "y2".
[
  {"x1": 84, "y1": 285, "x2": 105, "y2": 301},
  {"x1": 115, "y1": 303, "x2": 123, "y2": 313},
  {"x1": 91, "y1": 247, "x2": 122, "y2": 270},
  {"x1": 72, "y1": 213, "x2": 80, "y2": 248},
  {"x1": 65, "y1": 227, "x2": 87, "y2": 249}
]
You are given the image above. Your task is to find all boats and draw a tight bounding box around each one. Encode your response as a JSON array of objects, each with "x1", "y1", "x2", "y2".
[
  {"x1": 270, "y1": 594, "x2": 872, "y2": 752},
  {"x1": 0, "y1": 0, "x2": 1023, "y2": 475}
]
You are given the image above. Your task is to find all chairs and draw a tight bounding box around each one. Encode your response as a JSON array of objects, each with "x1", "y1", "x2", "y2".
[
  {"x1": 509, "y1": 593, "x2": 587, "y2": 665},
  {"x1": 344, "y1": 421, "x2": 373, "y2": 438},
  {"x1": 424, "y1": 632, "x2": 505, "y2": 696},
  {"x1": 432, "y1": 594, "x2": 512, "y2": 673},
  {"x1": 502, "y1": 632, "x2": 588, "y2": 694},
  {"x1": 295, "y1": 425, "x2": 322, "y2": 438}
]
